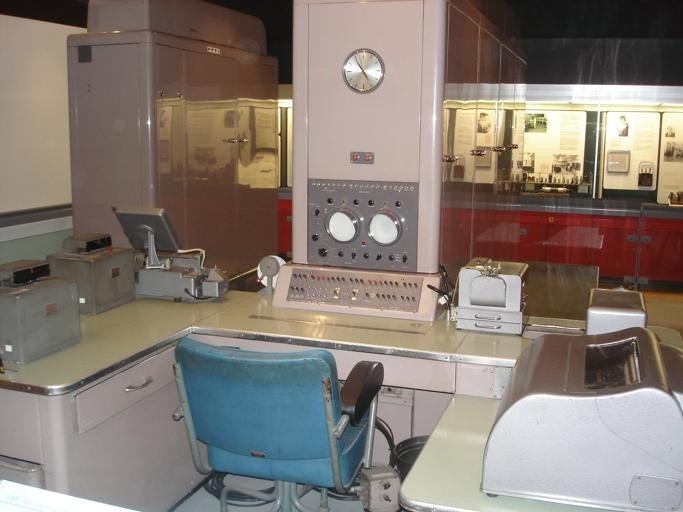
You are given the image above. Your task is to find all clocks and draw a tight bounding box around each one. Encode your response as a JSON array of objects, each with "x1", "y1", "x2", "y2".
[{"x1": 341, "y1": 47, "x2": 385, "y2": 93}]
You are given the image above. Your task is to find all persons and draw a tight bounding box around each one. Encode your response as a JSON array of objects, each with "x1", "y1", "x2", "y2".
[
  {"x1": 665, "y1": 126, "x2": 675, "y2": 137},
  {"x1": 618, "y1": 116, "x2": 628, "y2": 136},
  {"x1": 477, "y1": 112, "x2": 487, "y2": 133}
]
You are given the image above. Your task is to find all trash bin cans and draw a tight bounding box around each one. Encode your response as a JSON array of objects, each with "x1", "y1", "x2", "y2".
[{"x1": 394, "y1": 434, "x2": 431, "y2": 482}]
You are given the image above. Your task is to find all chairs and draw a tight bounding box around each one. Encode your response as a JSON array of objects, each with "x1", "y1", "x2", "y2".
[{"x1": 171, "y1": 337, "x2": 383, "y2": 511}]
